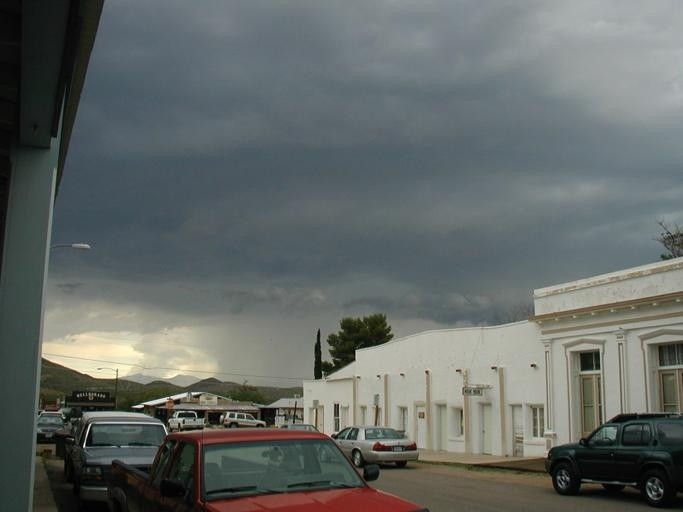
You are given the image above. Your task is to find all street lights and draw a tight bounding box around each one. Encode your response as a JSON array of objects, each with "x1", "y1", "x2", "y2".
[{"x1": 97, "y1": 365, "x2": 119, "y2": 408}]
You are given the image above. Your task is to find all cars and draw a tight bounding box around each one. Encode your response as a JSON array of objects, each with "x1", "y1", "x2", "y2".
[{"x1": 544, "y1": 412, "x2": 683, "y2": 506}]
